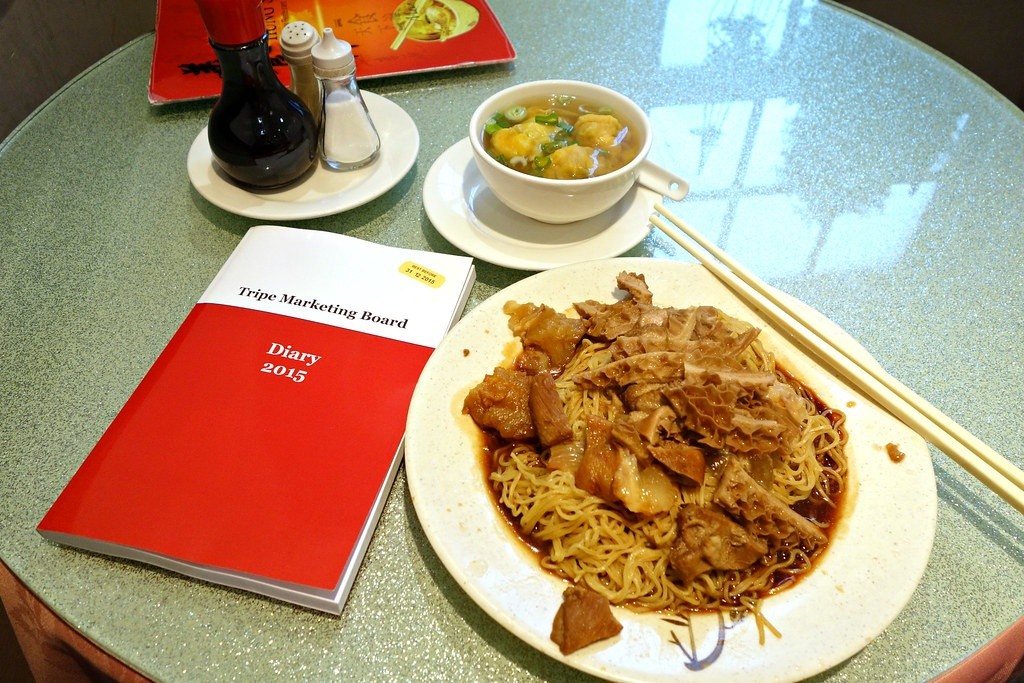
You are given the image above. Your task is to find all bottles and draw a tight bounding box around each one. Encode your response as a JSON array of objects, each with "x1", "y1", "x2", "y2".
[
  {"x1": 309, "y1": 27, "x2": 382, "y2": 170},
  {"x1": 197, "y1": 0, "x2": 319, "y2": 191},
  {"x1": 279, "y1": 21, "x2": 323, "y2": 125}
]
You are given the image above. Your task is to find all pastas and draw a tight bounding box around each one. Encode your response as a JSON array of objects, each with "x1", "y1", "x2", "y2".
[{"x1": 488, "y1": 335, "x2": 851, "y2": 644}]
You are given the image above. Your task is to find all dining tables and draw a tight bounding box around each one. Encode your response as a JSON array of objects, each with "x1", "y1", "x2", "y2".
[{"x1": 0, "y1": 0, "x2": 1024, "y2": 683}]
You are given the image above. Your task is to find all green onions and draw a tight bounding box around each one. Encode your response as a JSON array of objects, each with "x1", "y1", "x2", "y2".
[{"x1": 483, "y1": 97, "x2": 618, "y2": 177}]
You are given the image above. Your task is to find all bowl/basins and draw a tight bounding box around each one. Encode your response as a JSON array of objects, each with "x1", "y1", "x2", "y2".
[
  {"x1": 392, "y1": 0, "x2": 479, "y2": 43},
  {"x1": 468, "y1": 80, "x2": 652, "y2": 224}
]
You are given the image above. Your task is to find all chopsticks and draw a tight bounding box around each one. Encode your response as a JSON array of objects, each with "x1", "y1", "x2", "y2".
[
  {"x1": 647, "y1": 201, "x2": 1024, "y2": 511},
  {"x1": 390, "y1": 0, "x2": 426, "y2": 50}
]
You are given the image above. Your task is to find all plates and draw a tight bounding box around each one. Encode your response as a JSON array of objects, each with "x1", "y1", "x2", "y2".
[
  {"x1": 186, "y1": 90, "x2": 420, "y2": 219},
  {"x1": 406, "y1": 256, "x2": 939, "y2": 682},
  {"x1": 423, "y1": 137, "x2": 654, "y2": 270}
]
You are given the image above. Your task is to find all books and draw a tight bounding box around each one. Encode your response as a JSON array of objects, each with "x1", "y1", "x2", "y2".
[{"x1": 37, "y1": 226, "x2": 478, "y2": 616}]
